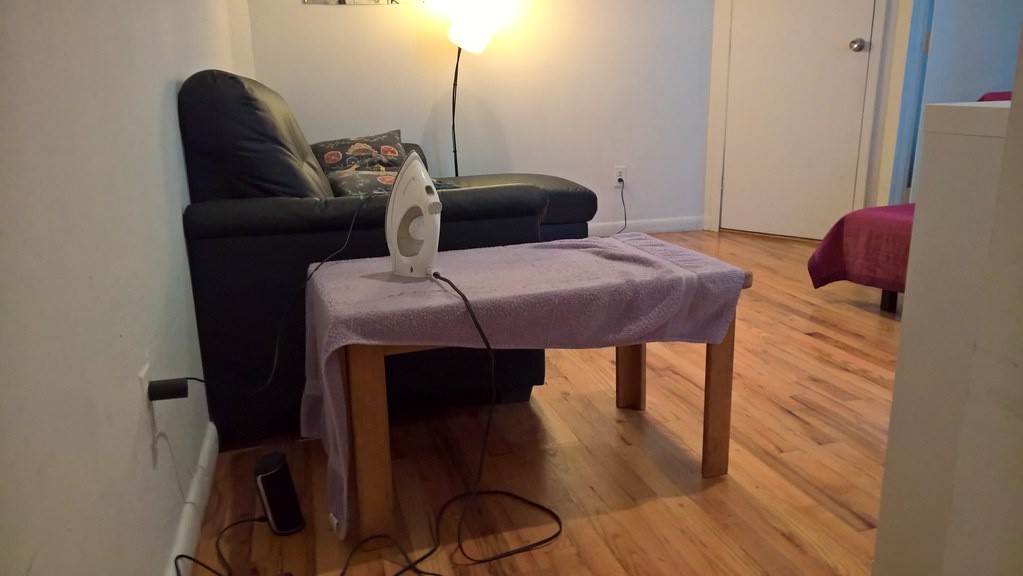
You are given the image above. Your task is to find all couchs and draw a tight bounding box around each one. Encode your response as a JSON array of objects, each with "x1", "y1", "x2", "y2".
[{"x1": 174, "y1": 69, "x2": 600, "y2": 455}]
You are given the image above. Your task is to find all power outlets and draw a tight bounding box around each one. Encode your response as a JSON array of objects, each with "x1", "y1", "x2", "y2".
[
  {"x1": 138, "y1": 361, "x2": 157, "y2": 420},
  {"x1": 613, "y1": 166, "x2": 626, "y2": 187}
]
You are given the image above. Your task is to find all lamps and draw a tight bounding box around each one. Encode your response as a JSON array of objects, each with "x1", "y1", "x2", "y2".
[{"x1": 449, "y1": 0, "x2": 509, "y2": 177}]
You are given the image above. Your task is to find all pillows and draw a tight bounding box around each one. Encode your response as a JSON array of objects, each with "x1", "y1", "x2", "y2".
[
  {"x1": 327, "y1": 166, "x2": 463, "y2": 197},
  {"x1": 308, "y1": 127, "x2": 407, "y2": 173}
]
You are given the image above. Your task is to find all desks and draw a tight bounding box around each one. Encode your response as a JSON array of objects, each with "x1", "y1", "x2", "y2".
[{"x1": 303, "y1": 230, "x2": 753, "y2": 550}]
